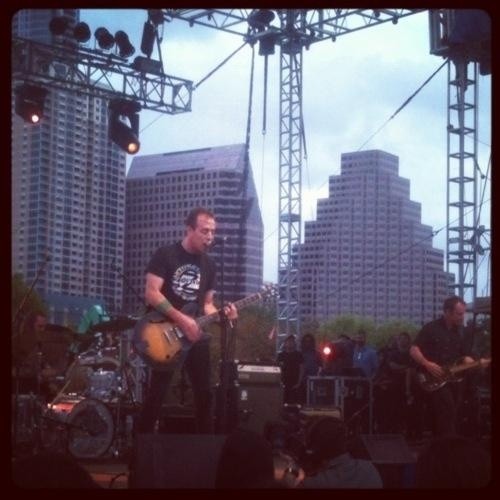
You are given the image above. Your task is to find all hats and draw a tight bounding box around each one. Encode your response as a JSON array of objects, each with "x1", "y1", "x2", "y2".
[{"x1": 283, "y1": 334, "x2": 295, "y2": 343}]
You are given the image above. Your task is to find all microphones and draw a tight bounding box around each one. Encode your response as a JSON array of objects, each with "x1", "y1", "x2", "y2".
[{"x1": 205, "y1": 237, "x2": 231, "y2": 249}]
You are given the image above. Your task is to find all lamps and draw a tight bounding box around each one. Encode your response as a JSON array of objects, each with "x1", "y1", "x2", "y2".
[
  {"x1": 108, "y1": 97, "x2": 141, "y2": 154},
  {"x1": 14, "y1": 83, "x2": 48, "y2": 124}
]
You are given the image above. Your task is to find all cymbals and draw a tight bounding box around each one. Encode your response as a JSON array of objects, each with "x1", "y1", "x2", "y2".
[
  {"x1": 90, "y1": 318, "x2": 137, "y2": 333},
  {"x1": 46, "y1": 324, "x2": 79, "y2": 336}
]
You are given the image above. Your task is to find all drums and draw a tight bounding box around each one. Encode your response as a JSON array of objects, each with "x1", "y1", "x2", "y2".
[
  {"x1": 63, "y1": 348, "x2": 129, "y2": 405},
  {"x1": 99, "y1": 346, "x2": 120, "y2": 357},
  {"x1": 12, "y1": 394, "x2": 46, "y2": 442},
  {"x1": 39, "y1": 393, "x2": 116, "y2": 462}
]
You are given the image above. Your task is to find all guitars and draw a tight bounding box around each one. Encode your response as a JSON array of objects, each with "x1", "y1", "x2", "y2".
[
  {"x1": 132, "y1": 282, "x2": 282, "y2": 371},
  {"x1": 415, "y1": 356, "x2": 492, "y2": 393}
]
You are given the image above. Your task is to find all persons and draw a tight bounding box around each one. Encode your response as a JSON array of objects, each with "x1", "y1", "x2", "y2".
[
  {"x1": 410, "y1": 296, "x2": 490, "y2": 423},
  {"x1": 12, "y1": 310, "x2": 59, "y2": 401},
  {"x1": 353, "y1": 330, "x2": 380, "y2": 380},
  {"x1": 300, "y1": 334, "x2": 322, "y2": 378},
  {"x1": 141, "y1": 206, "x2": 239, "y2": 436},
  {"x1": 388, "y1": 333, "x2": 413, "y2": 420},
  {"x1": 275, "y1": 335, "x2": 304, "y2": 404}
]
